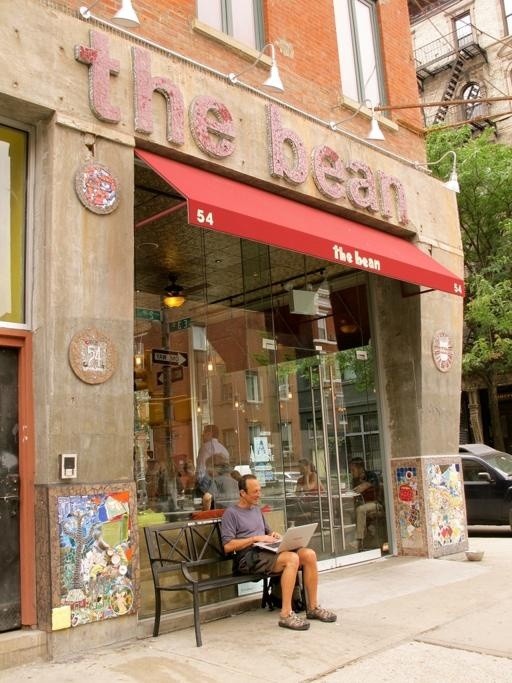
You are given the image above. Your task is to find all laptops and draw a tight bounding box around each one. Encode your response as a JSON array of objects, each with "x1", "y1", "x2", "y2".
[{"x1": 252, "y1": 521, "x2": 318, "y2": 553}]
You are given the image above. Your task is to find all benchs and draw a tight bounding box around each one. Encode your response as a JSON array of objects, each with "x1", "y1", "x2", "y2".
[{"x1": 144, "y1": 517, "x2": 308, "y2": 646}]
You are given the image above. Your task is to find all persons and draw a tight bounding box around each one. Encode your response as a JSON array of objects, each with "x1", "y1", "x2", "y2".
[
  {"x1": 219, "y1": 472, "x2": 337, "y2": 632},
  {"x1": 291, "y1": 454, "x2": 328, "y2": 518},
  {"x1": 230, "y1": 468, "x2": 241, "y2": 481},
  {"x1": 175, "y1": 454, "x2": 197, "y2": 494},
  {"x1": 200, "y1": 452, "x2": 244, "y2": 506},
  {"x1": 194, "y1": 422, "x2": 231, "y2": 484},
  {"x1": 344, "y1": 457, "x2": 385, "y2": 547}
]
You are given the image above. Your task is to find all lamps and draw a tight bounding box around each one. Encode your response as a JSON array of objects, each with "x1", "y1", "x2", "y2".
[
  {"x1": 229, "y1": 44, "x2": 284, "y2": 93},
  {"x1": 414, "y1": 151, "x2": 460, "y2": 193},
  {"x1": 329, "y1": 99, "x2": 385, "y2": 141},
  {"x1": 163, "y1": 291, "x2": 186, "y2": 308},
  {"x1": 80, "y1": 0, "x2": 140, "y2": 29}
]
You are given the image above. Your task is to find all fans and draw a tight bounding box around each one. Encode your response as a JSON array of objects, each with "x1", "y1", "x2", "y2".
[{"x1": 143, "y1": 271, "x2": 213, "y2": 294}]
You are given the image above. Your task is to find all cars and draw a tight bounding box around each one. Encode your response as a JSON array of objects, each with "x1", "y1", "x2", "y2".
[
  {"x1": 460, "y1": 442, "x2": 511, "y2": 529},
  {"x1": 276, "y1": 471, "x2": 301, "y2": 480}
]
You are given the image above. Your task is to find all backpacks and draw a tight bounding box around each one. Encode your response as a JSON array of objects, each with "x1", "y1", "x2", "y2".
[{"x1": 266, "y1": 575, "x2": 306, "y2": 613}]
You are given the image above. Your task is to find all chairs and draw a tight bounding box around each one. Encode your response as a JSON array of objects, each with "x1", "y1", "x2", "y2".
[{"x1": 137, "y1": 474, "x2": 388, "y2": 552}]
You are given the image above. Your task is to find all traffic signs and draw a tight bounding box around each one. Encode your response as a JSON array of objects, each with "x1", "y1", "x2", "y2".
[
  {"x1": 170, "y1": 316, "x2": 192, "y2": 332},
  {"x1": 150, "y1": 347, "x2": 190, "y2": 368},
  {"x1": 134, "y1": 306, "x2": 162, "y2": 322}
]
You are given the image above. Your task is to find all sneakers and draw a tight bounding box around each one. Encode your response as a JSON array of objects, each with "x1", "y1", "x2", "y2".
[
  {"x1": 306, "y1": 606, "x2": 336, "y2": 622},
  {"x1": 279, "y1": 611, "x2": 310, "y2": 630}
]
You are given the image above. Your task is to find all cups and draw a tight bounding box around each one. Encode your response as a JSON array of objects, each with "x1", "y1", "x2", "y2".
[{"x1": 340, "y1": 483, "x2": 346, "y2": 497}]
